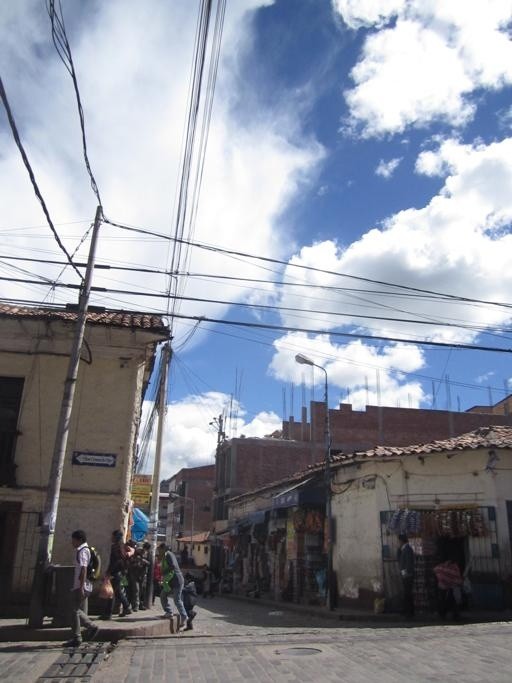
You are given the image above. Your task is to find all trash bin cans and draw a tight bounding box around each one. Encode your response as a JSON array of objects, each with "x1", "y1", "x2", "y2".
[{"x1": 51, "y1": 566, "x2": 89, "y2": 628}]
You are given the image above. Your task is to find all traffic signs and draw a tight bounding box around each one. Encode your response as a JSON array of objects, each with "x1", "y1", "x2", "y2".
[{"x1": 72, "y1": 452, "x2": 118, "y2": 469}]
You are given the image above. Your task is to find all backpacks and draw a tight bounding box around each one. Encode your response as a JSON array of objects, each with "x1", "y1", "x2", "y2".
[{"x1": 77, "y1": 547, "x2": 102, "y2": 581}]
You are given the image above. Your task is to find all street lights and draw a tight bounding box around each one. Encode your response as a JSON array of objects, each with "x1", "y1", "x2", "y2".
[
  {"x1": 292, "y1": 352, "x2": 336, "y2": 608},
  {"x1": 169, "y1": 491, "x2": 196, "y2": 566}
]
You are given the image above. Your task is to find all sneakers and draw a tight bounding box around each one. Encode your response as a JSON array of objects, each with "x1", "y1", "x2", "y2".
[
  {"x1": 99, "y1": 606, "x2": 146, "y2": 620},
  {"x1": 62, "y1": 639, "x2": 81, "y2": 647},
  {"x1": 88, "y1": 626, "x2": 99, "y2": 641},
  {"x1": 162, "y1": 610, "x2": 173, "y2": 618},
  {"x1": 178, "y1": 611, "x2": 196, "y2": 630}
]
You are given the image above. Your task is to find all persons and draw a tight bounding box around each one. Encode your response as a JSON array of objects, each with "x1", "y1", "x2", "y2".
[
  {"x1": 98, "y1": 530, "x2": 196, "y2": 632},
  {"x1": 200, "y1": 564, "x2": 215, "y2": 598},
  {"x1": 61, "y1": 530, "x2": 99, "y2": 647},
  {"x1": 397, "y1": 535, "x2": 415, "y2": 617}
]
